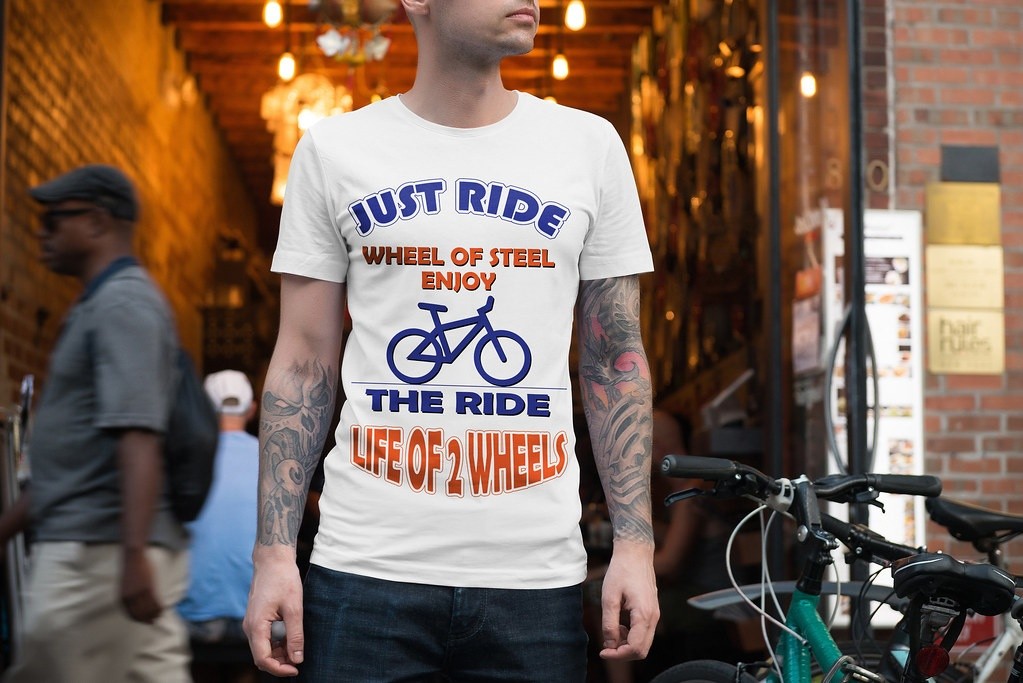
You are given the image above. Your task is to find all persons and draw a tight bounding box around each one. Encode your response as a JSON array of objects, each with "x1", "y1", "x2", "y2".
[
  {"x1": 237, "y1": 0, "x2": 663, "y2": 683},
  {"x1": 249, "y1": 328, "x2": 698, "y2": 680},
  {"x1": 179, "y1": 371, "x2": 260, "y2": 682},
  {"x1": 0, "y1": 163, "x2": 194, "y2": 683}
]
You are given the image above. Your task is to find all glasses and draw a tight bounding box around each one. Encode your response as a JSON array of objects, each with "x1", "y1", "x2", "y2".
[{"x1": 43, "y1": 208, "x2": 96, "y2": 233}]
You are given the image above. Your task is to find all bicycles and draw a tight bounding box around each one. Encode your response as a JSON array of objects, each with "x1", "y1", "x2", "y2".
[
  {"x1": 780, "y1": 495, "x2": 1022, "y2": 682},
  {"x1": 642, "y1": 454, "x2": 1017, "y2": 683}
]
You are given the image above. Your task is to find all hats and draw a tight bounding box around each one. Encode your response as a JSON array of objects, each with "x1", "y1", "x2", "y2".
[
  {"x1": 29, "y1": 164, "x2": 138, "y2": 221},
  {"x1": 203, "y1": 369, "x2": 253, "y2": 415}
]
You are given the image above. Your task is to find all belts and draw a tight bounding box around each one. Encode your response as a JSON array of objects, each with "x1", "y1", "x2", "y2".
[{"x1": 191, "y1": 618, "x2": 251, "y2": 642}]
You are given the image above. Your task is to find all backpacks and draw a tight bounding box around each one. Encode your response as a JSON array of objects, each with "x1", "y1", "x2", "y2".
[{"x1": 108, "y1": 276, "x2": 220, "y2": 521}]
